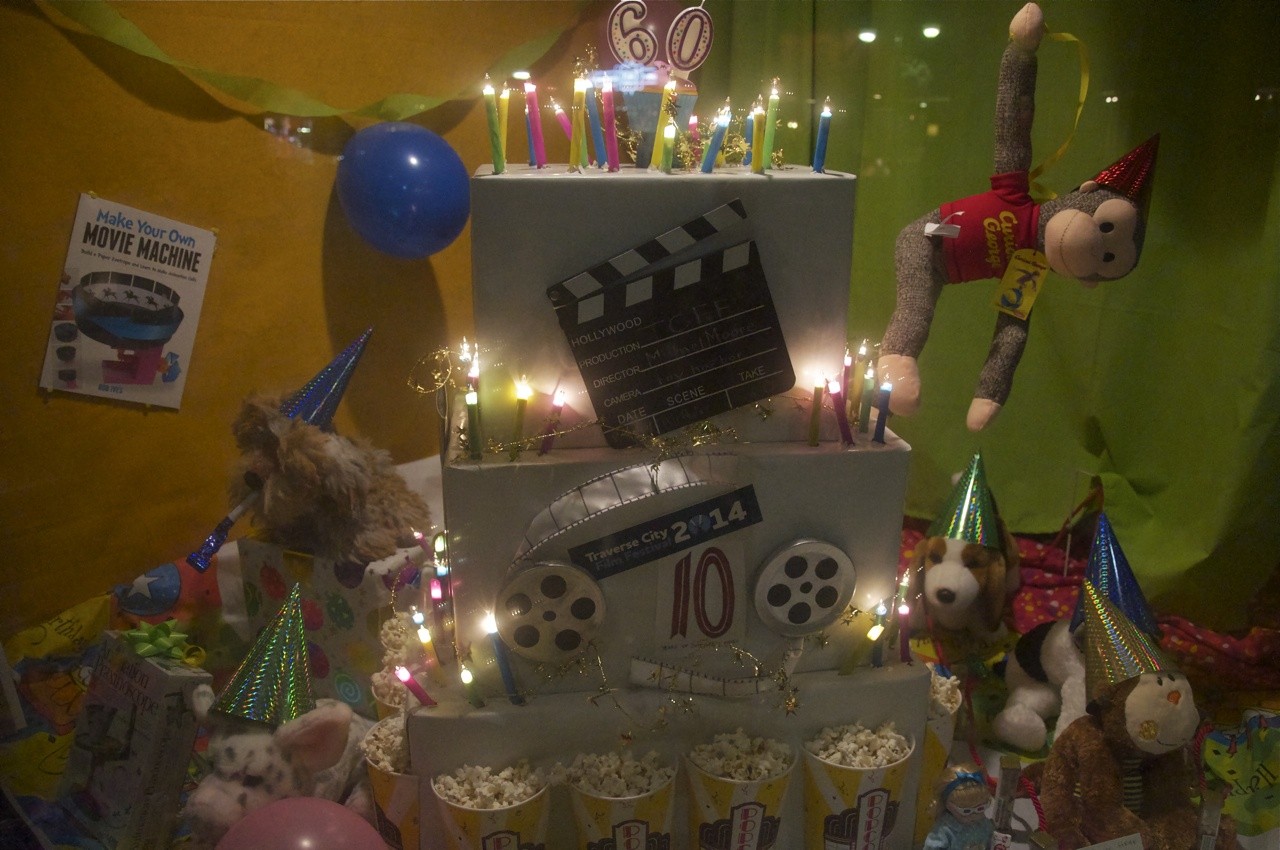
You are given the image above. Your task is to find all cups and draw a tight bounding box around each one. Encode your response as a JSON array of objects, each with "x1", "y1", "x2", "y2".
[
  {"x1": 428, "y1": 776, "x2": 551, "y2": 850},
  {"x1": 914, "y1": 674, "x2": 961, "y2": 850},
  {"x1": 569, "y1": 761, "x2": 678, "y2": 850},
  {"x1": 799, "y1": 732, "x2": 916, "y2": 850},
  {"x1": 364, "y1": 629, "x2": 420, "y2": 850},
  {"x1": 681, "y1": 742, "x2": 799, "y2": 850}
]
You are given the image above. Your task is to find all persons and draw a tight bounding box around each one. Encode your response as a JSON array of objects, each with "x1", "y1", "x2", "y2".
[{"x1": 921, "y1": 764, "x2": 1008, "y2": 850}]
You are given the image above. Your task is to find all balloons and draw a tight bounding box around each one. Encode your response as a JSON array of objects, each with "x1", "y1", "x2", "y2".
[
  {"x1": 336, "y1": 121, "x2": 470, "y2": 257},
  {"x1": 214, "y1": 797, "x2": 389, "y2": 850}
]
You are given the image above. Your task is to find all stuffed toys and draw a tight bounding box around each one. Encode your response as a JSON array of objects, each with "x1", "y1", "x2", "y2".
[
  {"x1": 184, "y1": 684, "x2": 377, "y2": 842},
  {"x1": 900, "y1": 522, "x2": 1020, "y2": 635},
  {"x1": 877, "y1": 1, "x2": 1163, "y2": 431},
  {"x1": 1041, "y1": 658, "x2": 1239, "y2": 850},
  {"x1": 991, "y1": 617, "x2": 1089, "y2": 750},
  {"x1": 228, "y1": 386, "x2": 431, "y2": 568}
]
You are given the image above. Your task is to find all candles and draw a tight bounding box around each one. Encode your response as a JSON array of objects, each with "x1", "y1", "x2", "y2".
[
  {"x1": 460, "y1": 341, "x2": 893, "y2": 458},
  {"x1": 482, "y1": 78, "x2": 832, "y2": 176},
  {"x1": 872, "y1": 569, "x2": 912, "y2": 667},
  {"x1": 395, "y1": 533, "x2": 522, "y2": 707}
]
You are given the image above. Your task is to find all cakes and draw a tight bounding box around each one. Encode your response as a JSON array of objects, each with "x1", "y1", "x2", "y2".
[{"x1": 353, "y1": 1, "x2": 965, "y2": 850}]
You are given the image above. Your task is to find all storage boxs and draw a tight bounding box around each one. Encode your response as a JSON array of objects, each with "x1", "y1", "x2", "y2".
[
  {"x1": 237, "y1": 530, "x2": 459, "y2": 723},
  {"x1": 61, "y1": 629, "x2": 215, "y2": 850}
]
